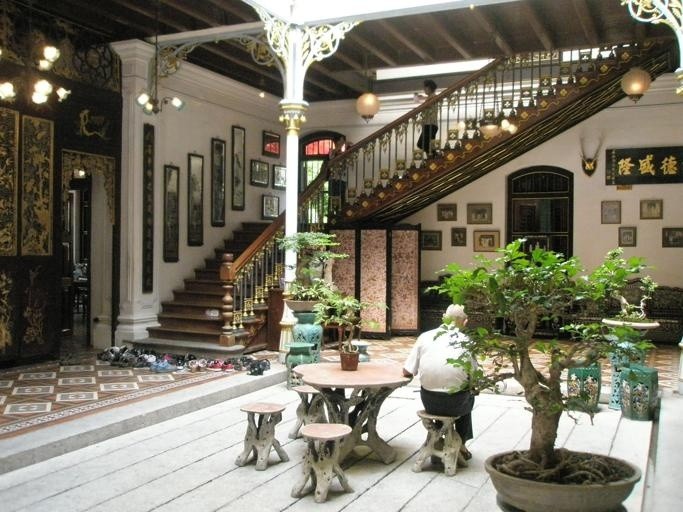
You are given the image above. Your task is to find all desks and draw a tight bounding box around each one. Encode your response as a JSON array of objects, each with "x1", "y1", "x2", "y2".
[
  {"x1": 73, "y1": 278, "x2": 88, "y2": 320},
  {"x1": 291, "y1": 363, "x2": 413, "y2": 466}
]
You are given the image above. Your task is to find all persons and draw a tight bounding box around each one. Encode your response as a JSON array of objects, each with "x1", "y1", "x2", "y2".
[
  {"x1": 416, "y1": 80, "x2": 438, "y2": 156},
  {"x1": 328, "y1": 134, "x2": 346, "y2": 214},
  {"x1": 402, "y1": 304, "x2": 479, "y2": 461}
]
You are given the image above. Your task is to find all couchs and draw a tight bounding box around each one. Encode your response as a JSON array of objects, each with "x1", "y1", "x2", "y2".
[{"x1": 569, "y1": 277, "x2": 683, "y2": 345}]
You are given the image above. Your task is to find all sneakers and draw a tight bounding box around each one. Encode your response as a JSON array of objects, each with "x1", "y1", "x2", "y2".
[
  {"x1": 95, "y1": 345, "x2": 271, "y2": 377},
  {"x1": 460, "y1": 447, "x2": 473, "y2": 462}
]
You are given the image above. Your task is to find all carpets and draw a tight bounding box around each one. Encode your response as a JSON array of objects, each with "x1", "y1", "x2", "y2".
[{"x1": 0, "y1": 349, "x2": 287, "y2": 491}]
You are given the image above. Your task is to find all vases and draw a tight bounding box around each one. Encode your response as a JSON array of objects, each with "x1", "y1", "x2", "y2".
[
  {"x1": 284, "y1": 342, "x2": 315, "y2": 369},
  {"x1": 292, "y1": 312, "x2": 323, "y2": 363},
  {"x1": 341, "y1": 340, "x2": 372, "y2": 363}
]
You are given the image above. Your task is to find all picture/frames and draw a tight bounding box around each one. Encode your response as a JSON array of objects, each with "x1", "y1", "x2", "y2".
[
  {"x1": 601, "y1": 199, "x2": 683, "y2": 247},
  {"x1": 164, "y1": 125, "x2": 287, "y2": 261},
  {"x1": 420, "y1": 202, "x2": 500, "y2": 252}
]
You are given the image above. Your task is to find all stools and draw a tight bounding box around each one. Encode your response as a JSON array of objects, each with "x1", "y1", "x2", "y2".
[
  {"x1": 567, "y1": 362, "x2": 600, "y2": 414},
  {"x1": 238, "y1": 385, "x2": 354, "y2": 503},
  {"x1": 412, "y1": 411, "x2": 467, "y2": 477},
  {"x1": 620, "y1": 362, "x2": 658, "y2": 421}
]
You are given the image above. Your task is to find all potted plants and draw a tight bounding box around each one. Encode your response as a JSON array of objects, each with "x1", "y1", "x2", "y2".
[
  {"x1": 589, "y1": 246, "x2": 660, "y2": 342},
  {"x1": 273, "y1": 231, "x2": 349, "y2": 312},
  {"x1": 424, "y1": 238, "x2": 655, "y2": 512},
  {"x1": 311, "y1": 290, "x2": 391, "y2": 371}
]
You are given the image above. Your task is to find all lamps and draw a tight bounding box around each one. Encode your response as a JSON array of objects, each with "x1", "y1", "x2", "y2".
[
  {"x1": 355, "y1": 51, "x2": 380, "y2": 124},
  {"x1": 454, "y1": 110, "x2": 517, "y2": 140},
  {"x1": 0, "y1": 0, "x2": 71, "y2": 106},
  {"x1": 136, "y1": 6, "x2": 185, "y2": 115},
  {"x1": 621, "y1": 64, "x2": 651, "y2": 103}
]
